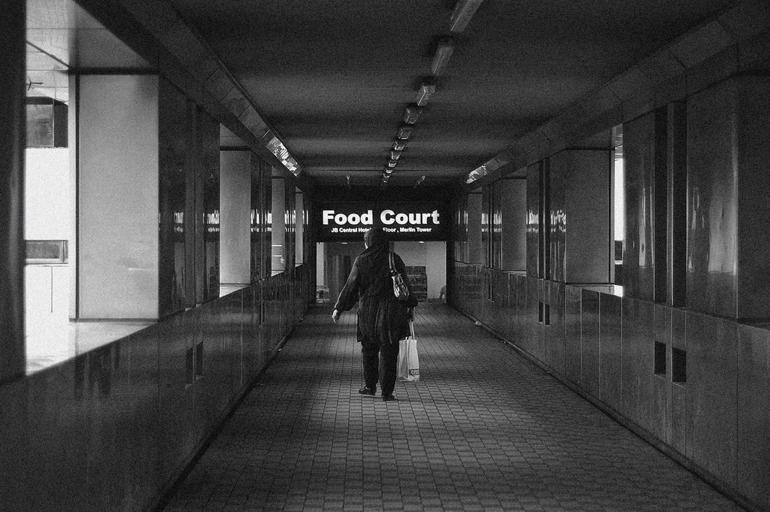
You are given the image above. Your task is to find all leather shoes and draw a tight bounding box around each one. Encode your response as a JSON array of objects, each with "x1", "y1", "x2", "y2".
[
  {"x1": 382, "y1": 393, "x2": 397, "y2": 402},
  {"x1": 359, "y1": 386, "x2": 375, "y2": 396}
]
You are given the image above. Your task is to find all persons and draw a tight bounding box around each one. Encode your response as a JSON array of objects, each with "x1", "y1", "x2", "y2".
[{"x1": 332, "y1": 227, "x2": 418, "y2": 400}]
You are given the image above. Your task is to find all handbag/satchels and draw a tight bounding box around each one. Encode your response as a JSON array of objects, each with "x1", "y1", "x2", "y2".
[
  {"x1": 388, "y1": 251, "x2": 410, "y2": 302},
  {"x1": 396, "y1": 319, "x2": 421, "y2": 383}
]
